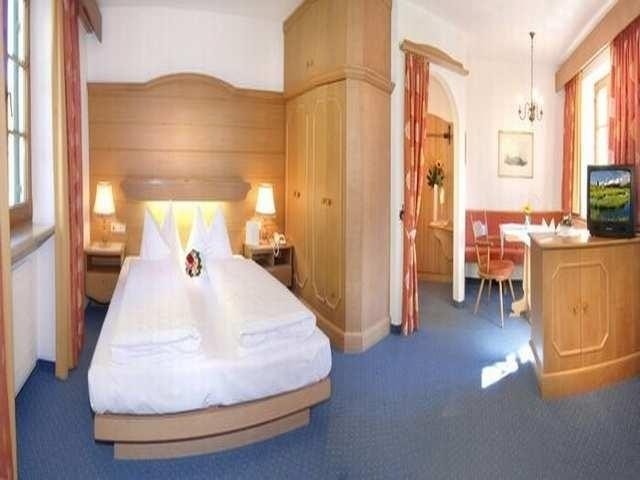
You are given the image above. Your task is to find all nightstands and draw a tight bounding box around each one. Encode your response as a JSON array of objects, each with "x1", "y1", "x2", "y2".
[
  {"x1": 85, "y1": 242, "x2": 126, "y2": 303},
  {"x1": 242, "y1": 242, "x2": 293, "y2": 288}
]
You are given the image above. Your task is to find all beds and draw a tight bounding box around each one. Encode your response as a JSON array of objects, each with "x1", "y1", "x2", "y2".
[{"x1": 87, "y1": 201, "x2": 332, "y2": 459}]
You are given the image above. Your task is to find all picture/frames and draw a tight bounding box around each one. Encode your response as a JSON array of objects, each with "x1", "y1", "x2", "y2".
[{"x1": 498, "y1": 130, "x2": 534, "y2": 178}]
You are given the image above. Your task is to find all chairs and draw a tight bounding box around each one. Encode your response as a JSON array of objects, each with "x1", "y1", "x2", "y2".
[{"x1": 469, "y1": 214, "x2": 516, "y2": 328}]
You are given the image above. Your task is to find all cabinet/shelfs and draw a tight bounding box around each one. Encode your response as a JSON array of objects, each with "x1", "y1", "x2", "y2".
[{"x1": 527, "y1": 232, "x2": 640, "y2": 399}]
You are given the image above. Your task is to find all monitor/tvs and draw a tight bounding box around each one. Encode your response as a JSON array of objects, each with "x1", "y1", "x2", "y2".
[{"x1": 586, "y1": 165, "x2": 636, "y2": 238}]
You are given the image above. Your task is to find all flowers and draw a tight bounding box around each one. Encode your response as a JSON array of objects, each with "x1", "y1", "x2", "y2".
[
  {"x1": 523, "y1": 205, "x2": 533, "y2": 214},
  {"x1": 425, "y1": 159, "x2": 446, "y2": 188},
  {"x1": 185, "y1": 250, "x2": 202, "y2": 278}
]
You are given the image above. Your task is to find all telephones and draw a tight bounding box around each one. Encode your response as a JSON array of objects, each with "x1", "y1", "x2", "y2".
[{"x1": 273, "y1": 232, "x2": 286, "y2": 244}]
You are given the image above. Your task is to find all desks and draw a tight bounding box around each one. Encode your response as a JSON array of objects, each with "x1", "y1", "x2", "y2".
[
  {"x1": 499, "y1": 222, "x2": 591, "y2": 317},
  {"x1": 429, "y1": 221, "x2": 452, "y2": 263}
]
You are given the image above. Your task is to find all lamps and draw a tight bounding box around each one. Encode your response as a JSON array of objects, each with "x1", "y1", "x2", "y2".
[
  {"x1": 94, "y1": 181, "x2": 116, "y2": 247},
  {"x1": 255, "y1": 183, "x2": 277, "y2": 244},
  {"x1": 514, "y1": 32, "x2": 544, "y2": 122}
]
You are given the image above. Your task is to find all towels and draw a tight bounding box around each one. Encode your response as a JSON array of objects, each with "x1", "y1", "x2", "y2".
[
  {"x1": 555, "y1": 223, "x2": 561, "y2": 232},
  {"x1": 541, "y1": 216, "x2": 556, "y2": 231}
]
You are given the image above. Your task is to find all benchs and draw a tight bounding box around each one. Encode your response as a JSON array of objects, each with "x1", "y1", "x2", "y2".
[{"x1": 465, "y1": 208, "x2": 564, "y2": 280}]
